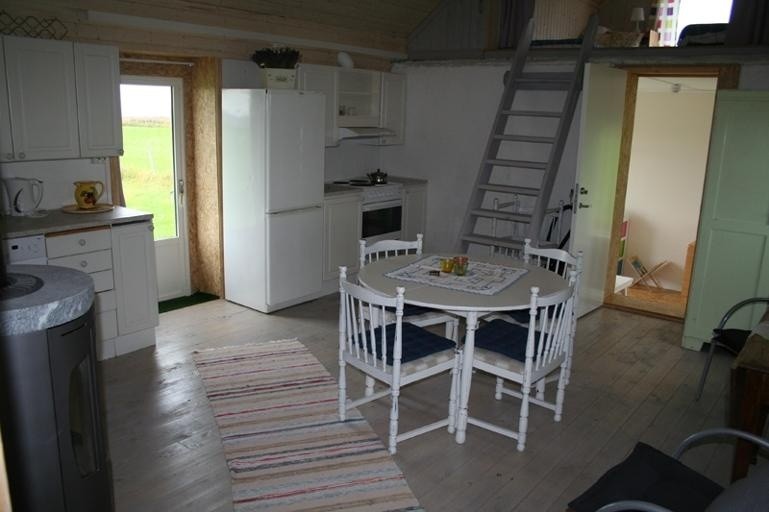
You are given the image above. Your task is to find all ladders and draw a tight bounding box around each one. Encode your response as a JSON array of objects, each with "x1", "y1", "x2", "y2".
[{"x1": 455, "y1": 14, "x2": 598, "y2": 261}]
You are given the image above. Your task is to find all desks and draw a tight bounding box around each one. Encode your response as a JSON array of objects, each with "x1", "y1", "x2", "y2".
[{"x1": 725, "y1": 306, "x2": 768, "y2": 484}]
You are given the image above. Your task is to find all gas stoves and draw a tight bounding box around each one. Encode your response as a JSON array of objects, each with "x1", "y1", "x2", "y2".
[{"x1": 335, "y1": 178, "x2": 405, "y2": 204}]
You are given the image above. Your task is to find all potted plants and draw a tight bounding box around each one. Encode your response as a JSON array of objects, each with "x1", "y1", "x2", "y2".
[{"x1": 251, "y1": 47, "x2": 300, "y2": 90}]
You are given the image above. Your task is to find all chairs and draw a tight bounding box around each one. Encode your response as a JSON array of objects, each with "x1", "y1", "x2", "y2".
[
  {"x1": 565, "y1": 427, "x2": 769, "y2": 512},
  {"x1": 455, "y1": 271, "x2": 578, "y2": 453},
  {"x1": 336, "y1": 265, "x2": 459, "y2": 453},
  {"x1": 358, "y1": 232, "x2": 459, "y2": 399},
  {"x1": 696, "y1": 296, "x2": 769, "y2": 402},
  {"x1": 470, "y1": 239, "x2": 584, "y2": 386}
]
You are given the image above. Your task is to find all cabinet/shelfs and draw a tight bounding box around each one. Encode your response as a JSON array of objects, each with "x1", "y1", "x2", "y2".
[
  {"x1": 0, "y1": 33, "x2": 81, "y2": 163},
  {"x1": 112, "y1": 217, "x2": 161, "y2": 358},
  {"x1": 74, "y1": 40, "x2": 124, "y2": 159},
  {"x1": 402, "y1": 184, "x2": 426, "y2": 253},
  {"x1": 337, "y1": 66, "x2": 381, "y2": 127},
  {"x1": 1, "y1": 226, "x2": 117, "y2": 362},
  {"x1": 616, "y1": 218, "x2": 628, "y2": 275},
  {"x1": 323, "y1": 192, "x2": 360, "y2": 297},
  {"x1": 357, "y1": 70, "x2": 405, "y2": 146},
  {"x1": 297, "y1": 64, "x2": 338, "y2": 148}
]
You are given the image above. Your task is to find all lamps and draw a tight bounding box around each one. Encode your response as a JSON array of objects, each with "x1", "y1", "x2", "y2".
[{"x1": 631, "y1": 6, "x2": 645, "y2": 33}]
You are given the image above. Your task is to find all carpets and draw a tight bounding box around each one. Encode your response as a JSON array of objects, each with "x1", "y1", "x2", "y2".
[{"x1": 192, "y1": 336, "x2": 427, "y2": 511}]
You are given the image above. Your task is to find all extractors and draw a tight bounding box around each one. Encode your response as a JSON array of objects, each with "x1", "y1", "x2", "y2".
[{"x1": 337, "y1": 126, "x2": 396, "y2": 140}]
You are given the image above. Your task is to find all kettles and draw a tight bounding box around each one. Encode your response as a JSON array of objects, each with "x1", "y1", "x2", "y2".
[{"x1": 3, "y1": 176, "x2": 44, "y2": 216}]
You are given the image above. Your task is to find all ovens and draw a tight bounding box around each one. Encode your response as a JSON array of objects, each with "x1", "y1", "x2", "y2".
[{"x1": 360, "y1": 199, "x2": 402, "y2": 246}]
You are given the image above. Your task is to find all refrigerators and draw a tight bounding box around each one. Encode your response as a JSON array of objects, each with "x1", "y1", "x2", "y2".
[{"x1": 222, "y1": 86, "x2": 325, "y2": 308}]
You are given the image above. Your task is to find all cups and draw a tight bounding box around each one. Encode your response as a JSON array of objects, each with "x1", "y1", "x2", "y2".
[{"x1": 438, "y1": 255, "x2": 468, "y2": 276}]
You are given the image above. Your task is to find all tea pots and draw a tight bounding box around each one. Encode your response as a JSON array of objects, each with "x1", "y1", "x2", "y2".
[{"x1": 73, "y1": 179, "x2": 104, "y2": 208}]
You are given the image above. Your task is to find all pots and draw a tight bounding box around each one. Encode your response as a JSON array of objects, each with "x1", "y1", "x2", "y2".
[{"x1": 367, "y1": 168, "x2": 388, "y2": 183}]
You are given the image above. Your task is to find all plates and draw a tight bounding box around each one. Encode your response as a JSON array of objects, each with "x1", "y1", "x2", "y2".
[{"x1": 61, "y1": 203, "x2": 113, "y2": 214}]
